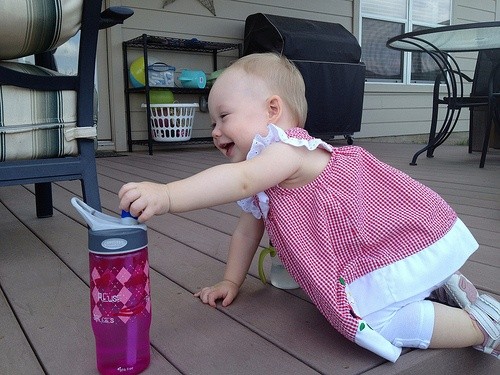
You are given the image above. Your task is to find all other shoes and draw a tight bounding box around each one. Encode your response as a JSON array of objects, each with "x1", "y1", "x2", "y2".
[
  {"x1": 446, "y1": 271, "x2": 478, "y2": 308},
  {"x1": 467, "y1": 294, "x2": 500, "y2": 359}
]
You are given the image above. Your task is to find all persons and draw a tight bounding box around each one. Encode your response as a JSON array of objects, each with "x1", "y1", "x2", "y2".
[{"x1": 117, "y1": 51, "x2": 500, "y2": 358}]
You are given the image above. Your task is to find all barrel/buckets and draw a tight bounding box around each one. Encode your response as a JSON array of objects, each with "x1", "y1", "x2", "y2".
[{"x1": 174, "y1": 68, "x2": 206, "y2": 88}]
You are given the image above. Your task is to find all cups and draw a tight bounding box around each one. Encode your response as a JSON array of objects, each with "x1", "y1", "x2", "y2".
[{"x1": 71, "y1": 197, "x2": 151, "y2": 374}]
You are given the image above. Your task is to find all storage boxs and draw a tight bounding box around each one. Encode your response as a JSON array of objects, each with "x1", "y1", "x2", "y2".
[{"x1": 147, "y1": 62, "x2": 176, "y2": 87}]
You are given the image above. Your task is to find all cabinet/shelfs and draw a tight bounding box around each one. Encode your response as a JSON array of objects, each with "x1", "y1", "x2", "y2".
[{"x1": 122, "y1": 33, "x2": 243, "y2": 156}]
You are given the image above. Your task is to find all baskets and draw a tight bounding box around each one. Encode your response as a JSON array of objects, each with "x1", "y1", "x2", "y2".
[{"x1": 139, "y1": 102, "x2": 200, "y2": 142}]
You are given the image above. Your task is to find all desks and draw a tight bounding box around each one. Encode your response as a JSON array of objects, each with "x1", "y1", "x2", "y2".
[{"x1": 386, "y1": 20, "x2": 500, "y2": 169}]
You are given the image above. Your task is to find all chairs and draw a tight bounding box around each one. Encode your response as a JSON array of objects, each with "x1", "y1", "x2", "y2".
[{"x1": 0, "y1": 0, "x2": 133, "y2": 229}]
